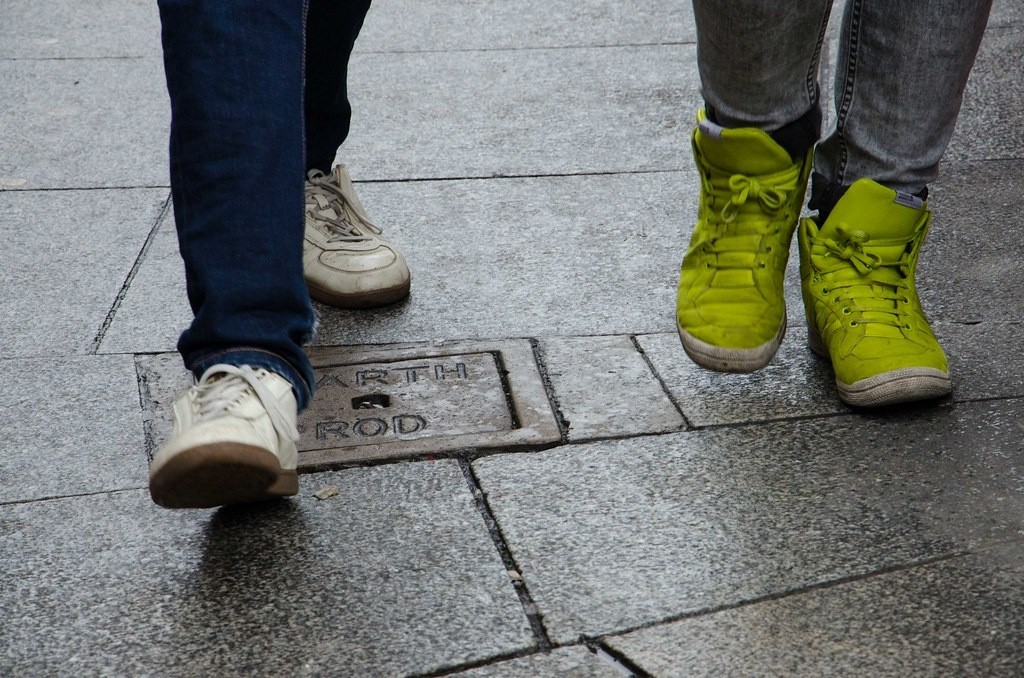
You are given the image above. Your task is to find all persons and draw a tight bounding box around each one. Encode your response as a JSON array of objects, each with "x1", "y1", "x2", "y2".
[
  {"x1": 151, "y1": 0, "x2": 412, "y2": 509},
  {"x1": 676, "y1": 0, "x2": 993, "y2": 406}
]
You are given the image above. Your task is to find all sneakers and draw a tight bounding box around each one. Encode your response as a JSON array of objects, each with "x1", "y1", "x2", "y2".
[
  {"x1": 796, "y1": 178, "x2": 951, "y2": 408},
  {"x1": 679, "y1": 108, "x2": 813, "y2": 373},
  {"x1": 300, "y1": 162, "x2": 411, "y2": 308},
  {"x1": 149, "y1": 355, "x2": 298, "y2": 509}
]
